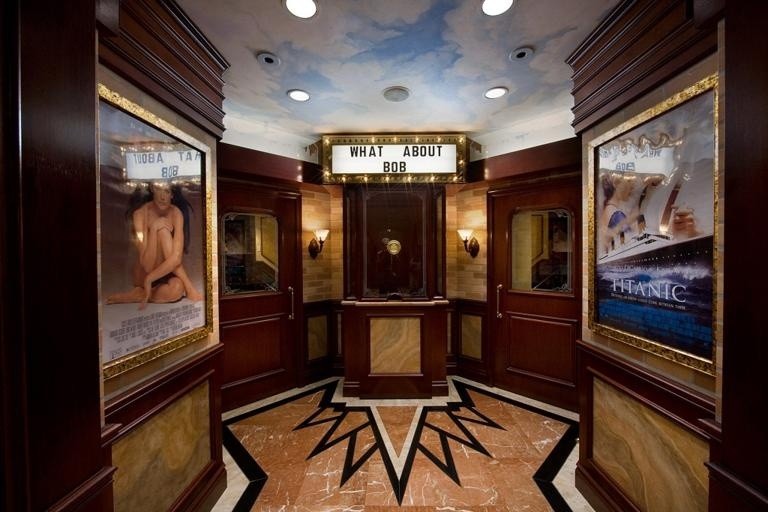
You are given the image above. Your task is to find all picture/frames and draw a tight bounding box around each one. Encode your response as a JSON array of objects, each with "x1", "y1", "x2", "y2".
[
  {"x1": 586, "y1": 72, "x2": 721, "y2": 378},
  {"x1": 98, "y1": 83, "x2": 213, "y2": 382}
]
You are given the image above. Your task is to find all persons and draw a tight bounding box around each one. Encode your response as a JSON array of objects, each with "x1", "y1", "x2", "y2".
[
  {"x1": 103, "y1": 177, "x2": 203, "y2": 312},
  {"x1": 597, "y1": 167, "x2": 643, "y2": 256},
  {"x1": 670, "y1": 205, "x2": 701, "y2": 241}
]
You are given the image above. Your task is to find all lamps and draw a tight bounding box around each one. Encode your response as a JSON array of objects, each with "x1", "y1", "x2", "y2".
[
  {"x1": 456, "y1": 229, "x2": 479, "y2": 258},
  {"x1": 309, "y1": 229, "x2": 329, "y2": 259},
  {"x1": 281, "y1": 0, "x2": 318, "y2": 22},
  {"x1": 479, "y1": 0, "x2": 519, "y2": 19},
  {"x1": 288, "y1": 89, "x2": 311, "y2": 102},
  {"x1": 482, "y1": 87, "x2": 508, "y2": 100}
]
